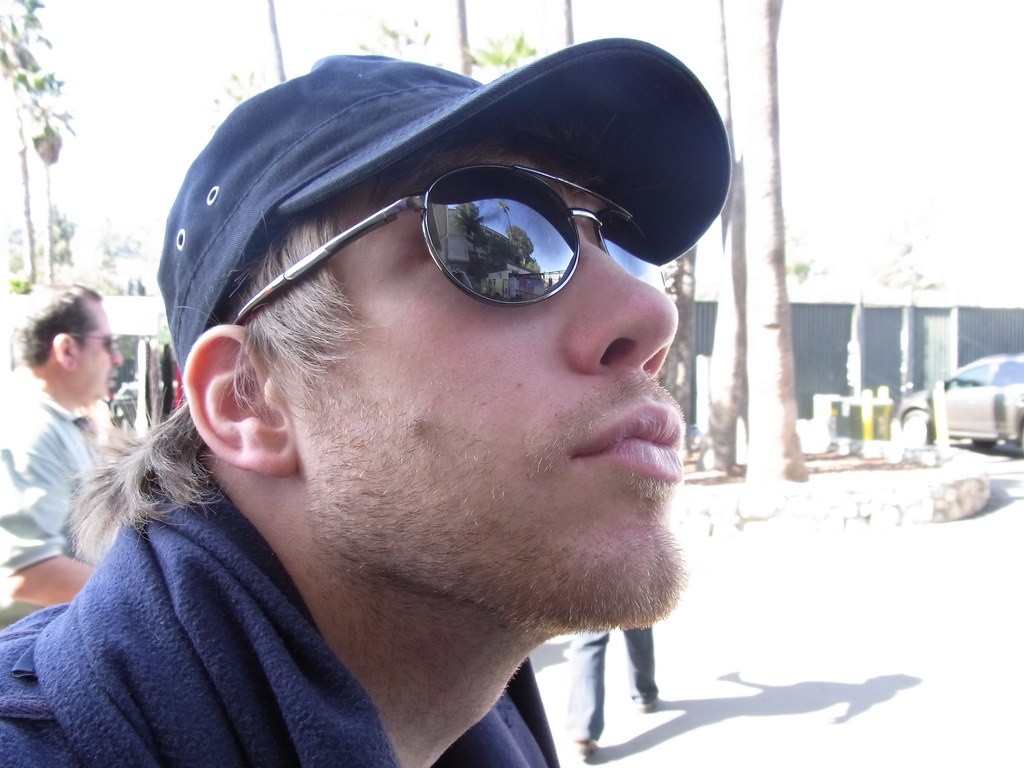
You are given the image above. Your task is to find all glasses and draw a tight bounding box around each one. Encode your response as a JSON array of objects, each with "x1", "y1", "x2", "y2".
[
  {"x1": 70, "y1": 331, "x2": 114, "y2": 353},
  {"x1": 229, "y1": 162, "x2": 666, "y2": 324}
]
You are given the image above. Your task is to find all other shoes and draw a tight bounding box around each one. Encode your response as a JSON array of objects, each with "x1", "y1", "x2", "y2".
[
  {"x1": 575, "y1": 741, "x2": 599, "y2": 762},
  {"x1": 635, "y1": 693, "x2": 657, "y2": 714}
]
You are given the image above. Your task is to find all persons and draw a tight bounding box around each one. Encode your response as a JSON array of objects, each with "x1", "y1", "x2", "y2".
[
  {"x1": 1, "y1": 281, "x2": 122, "y2": 635},
  {"x1": 567, "y1": 624, "x2": 662, "y2": 756},
  {"x1": 0, "y1": 35, "x2": 737, "y2": 767}
]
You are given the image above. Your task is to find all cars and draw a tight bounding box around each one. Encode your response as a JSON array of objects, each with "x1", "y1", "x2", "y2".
[{"x1": 896, "y1": 350, "x2": 1024, "y2": 454}]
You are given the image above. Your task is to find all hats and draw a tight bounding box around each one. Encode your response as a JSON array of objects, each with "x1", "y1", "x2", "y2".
[{"x1": 155, "y1": 35, "x2": 732, "y2": 383}]
